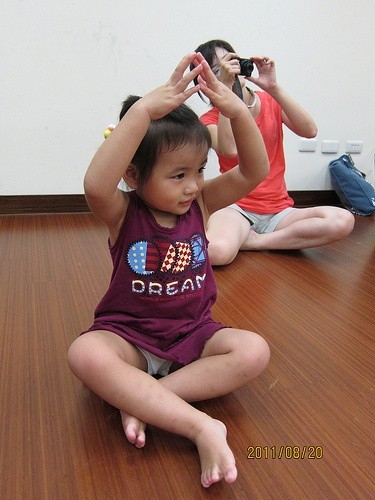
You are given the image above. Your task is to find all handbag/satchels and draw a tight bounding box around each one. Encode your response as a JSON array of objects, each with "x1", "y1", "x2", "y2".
[{"x1": 328, "y1": 154, "x2": 375, "y2": 216}]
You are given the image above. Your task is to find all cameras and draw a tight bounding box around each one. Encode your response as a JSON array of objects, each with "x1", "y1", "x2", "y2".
[{"x1": 231, "y1": 57, "x2": 254, "y2": 77}]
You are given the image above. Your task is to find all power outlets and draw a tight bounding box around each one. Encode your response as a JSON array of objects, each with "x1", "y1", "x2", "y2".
[{"x1": 346, "y1": 140, "x2": 364, "y2": 154}]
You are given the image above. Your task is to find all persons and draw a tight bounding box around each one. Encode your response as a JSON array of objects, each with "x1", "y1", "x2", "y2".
[
  {"x1": 66, "y1": 52, "x2": 271, "y2": 487},
  {"x1": 189, "y1": 40, "x2": 355, "y2": 265}
]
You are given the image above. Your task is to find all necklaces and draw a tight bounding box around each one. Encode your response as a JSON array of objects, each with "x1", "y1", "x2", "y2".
[{"x1": 244, "y1": 86, "x2": 258, "y2": 109}]
